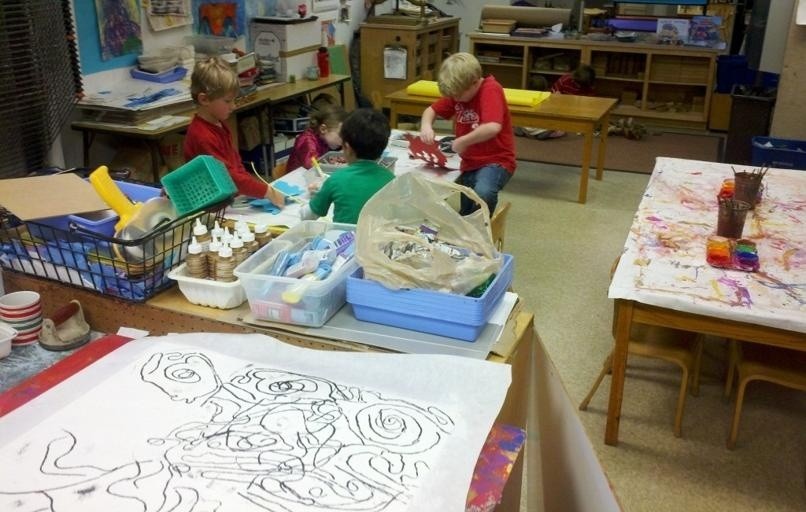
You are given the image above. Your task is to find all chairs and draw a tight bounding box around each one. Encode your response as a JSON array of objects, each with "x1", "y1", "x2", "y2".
[
  {"x1": 579, "y1": 252, "x2": 701, "y2": 434},
  {"x1": 487, "y1": 202, "x2": 512, "y2": 255},
  {"x1": 721, "y1": 335, "x2": 805, "y2": 450}
]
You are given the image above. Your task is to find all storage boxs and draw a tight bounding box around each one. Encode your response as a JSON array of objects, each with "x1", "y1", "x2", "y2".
[
  {"x1": 0, "y1": 185, "x2": 235, "y2": 306},
  {"x1": 747, "y1": 135, "x2": 806, "y2": 171},
  {"x1": 717, "y1": 49, "x2": 747, "y2": 86},
  {"x1": 168, "y1": 223, "x2": 358, "y2": 328},
  {"x1": 344, "y1": 249, "x2": 513, "y2": 340},
  {"x1": 180, "y1": 12, "x2": 324, "y2": 84}
]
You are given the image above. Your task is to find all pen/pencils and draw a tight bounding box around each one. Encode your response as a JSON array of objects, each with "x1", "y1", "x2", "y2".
[
  {"x1": 731, "y1": 162, "x2": 771, "y2": 176},
  {"x1": 312, "y1": 157, "x2": 326, "y2": 178}
]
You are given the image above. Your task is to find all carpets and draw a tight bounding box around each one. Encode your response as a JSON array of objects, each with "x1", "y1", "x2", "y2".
[{"x1": 512, "y1": 114, "x2": 721, "y2": 175}]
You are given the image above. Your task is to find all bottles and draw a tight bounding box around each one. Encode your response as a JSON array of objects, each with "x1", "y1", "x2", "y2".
[
  {"x1": 187, "y1": 216, "x2": 275, "y2": 282},
  {"x1": 733, "y1": 238, "x2": 762, "y2": 270},
  {"x1": 720, "y1": 176, "x2": 735, "y2": 200},
  {"x1": 706, "y1": 235, "x2": 732, "y2": 268},
  {"x1": 317, "y1": 46, "x2": 331, "y2": 79}
]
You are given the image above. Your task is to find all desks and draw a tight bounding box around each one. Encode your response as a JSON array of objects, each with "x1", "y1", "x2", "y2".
[
  {"x1": 600, "y1": 154, "x2": 806, "y2": 448},
  {"x1": 258, "y1": 68, "x2": 352, "y2": 174},
  {"x1": 66, "y1": 96, "x2": 271, "y2": 184},
  {"x1": 381, "y1": 80, "x2": 618, "y2": 205}
]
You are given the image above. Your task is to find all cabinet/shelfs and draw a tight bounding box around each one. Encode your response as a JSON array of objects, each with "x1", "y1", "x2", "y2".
[
  {"x1": 464, "y1": 24, "x2": 713, "y2": 133},
  {"x1": 355, "y1": 14, "x2": 461, "y2": 114},
  {"x1": 1, "y1": 224, "x2": 534, "y2": 511}
]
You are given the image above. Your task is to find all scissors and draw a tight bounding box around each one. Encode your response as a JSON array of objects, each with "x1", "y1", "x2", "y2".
[{"x1": 436, "y1": 140, "x2": 454, "y2": 151}]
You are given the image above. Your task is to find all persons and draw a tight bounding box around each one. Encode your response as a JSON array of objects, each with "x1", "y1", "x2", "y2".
[
  {"x1": 182, "y1": 58, "x2": 285, "y2": 211},
  {"x1": 417, "y1": 51, "x2": 516, "y2": 223},
  {"x1": 548, "y1": 64, "x2": 596, "y2": 98},
  {"x1": 286, "y1": 105, "x2": 344, "y2": 170},
  {"x1": 302, "y1": 109, "x2": 396, "y2": 224}
]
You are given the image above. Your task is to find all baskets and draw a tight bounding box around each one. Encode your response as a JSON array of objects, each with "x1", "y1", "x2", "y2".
[
  {"x1": 160, "y1": 153, "x2": 238, "y2": 217},
  {"x1": 0, "y1": 169, "x2": 227, "y2": 303}
]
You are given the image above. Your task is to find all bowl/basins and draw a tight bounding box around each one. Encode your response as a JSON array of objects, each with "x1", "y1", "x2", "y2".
[
  {"x1": 585, "y1": 31, "x2": 612, "y2": 42},
  {"x1": 616, "y1": 32, "x2": 638, "y2": 43}
]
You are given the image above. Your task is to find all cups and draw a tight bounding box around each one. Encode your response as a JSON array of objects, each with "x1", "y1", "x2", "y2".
[
  {"x1": 0, "y1": 289, "x2": 42, "y2": 347},
  {"x1": 736, "y1": 170, "x2": 761, "y2": 209},
  {"x1": 307, "y1": 65, "x2": 320, "y2": 81},
  {"x1": 718, "y1": 200, "x2": 751, "y2": 237}
]
